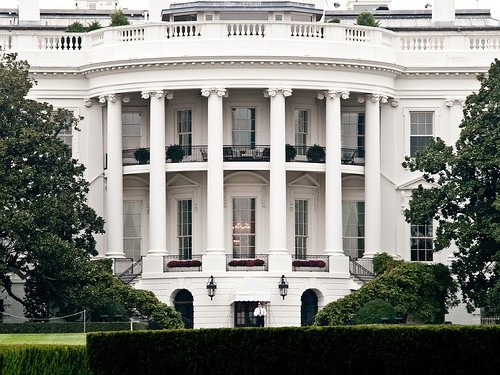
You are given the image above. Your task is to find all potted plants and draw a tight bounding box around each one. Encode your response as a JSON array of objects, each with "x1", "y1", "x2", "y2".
[
  {"x1": 134, "y1": 148, "x2": 150, "y2": 164},
  {"x1": 306, "y1": 144, "x2": 326, "y2": 163},
  {"x1": 285, "y1": 144, "x2": 297, "y2": 162},
  {"x1": 166, "y1": 144, "x2": 185, "y2": 163}
]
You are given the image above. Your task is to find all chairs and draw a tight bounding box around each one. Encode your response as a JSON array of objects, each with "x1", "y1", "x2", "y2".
[
  {"x1": 261, "y1": 148, "x2": 270, "y2": 162},
  {"x1": 200, "y1": 149, "x2": 208, "y2": 162},
  {"x1": 223, "y1": 147, "x2": 233, "y2": 161}
]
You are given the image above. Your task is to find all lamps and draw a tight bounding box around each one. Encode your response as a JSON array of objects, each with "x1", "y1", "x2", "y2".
[
  {"x1": 206, "y1": 275, "x2": 216, "y2": 300},
  {"x1": 279, "y1": 275, "x2": 289, "y2": 300}
]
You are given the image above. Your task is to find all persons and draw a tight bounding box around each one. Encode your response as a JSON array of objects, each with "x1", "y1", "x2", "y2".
[{"x1": 253, "y1": 302, "x2": 267, "y2": 327}]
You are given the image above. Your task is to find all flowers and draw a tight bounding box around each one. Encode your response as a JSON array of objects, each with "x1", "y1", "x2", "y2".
[
  {"x1": 228, "y1": 259, "x2": 264, "y2": 266},
  {"x1": 167, "y1": 260, "x2": 201, "y2": 267},
  {"x1": 292, "y1": 260, "x2": 326, "y2": 268}
]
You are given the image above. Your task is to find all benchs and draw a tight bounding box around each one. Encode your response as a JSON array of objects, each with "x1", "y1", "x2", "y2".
[{"x1": 341, "y1": 149, "x2": 358, "y2": 164}]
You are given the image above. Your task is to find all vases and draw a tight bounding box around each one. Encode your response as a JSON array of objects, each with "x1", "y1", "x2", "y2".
[
  {"x1": 294, "y1": 267, "x2": 325, "y2": 272},
  {"x1": 227, "y1": 265, "x2": 265, "y2": 271},
  {"x1": 167, "y1": 267, "x2": 200, "y2": 272}
]
